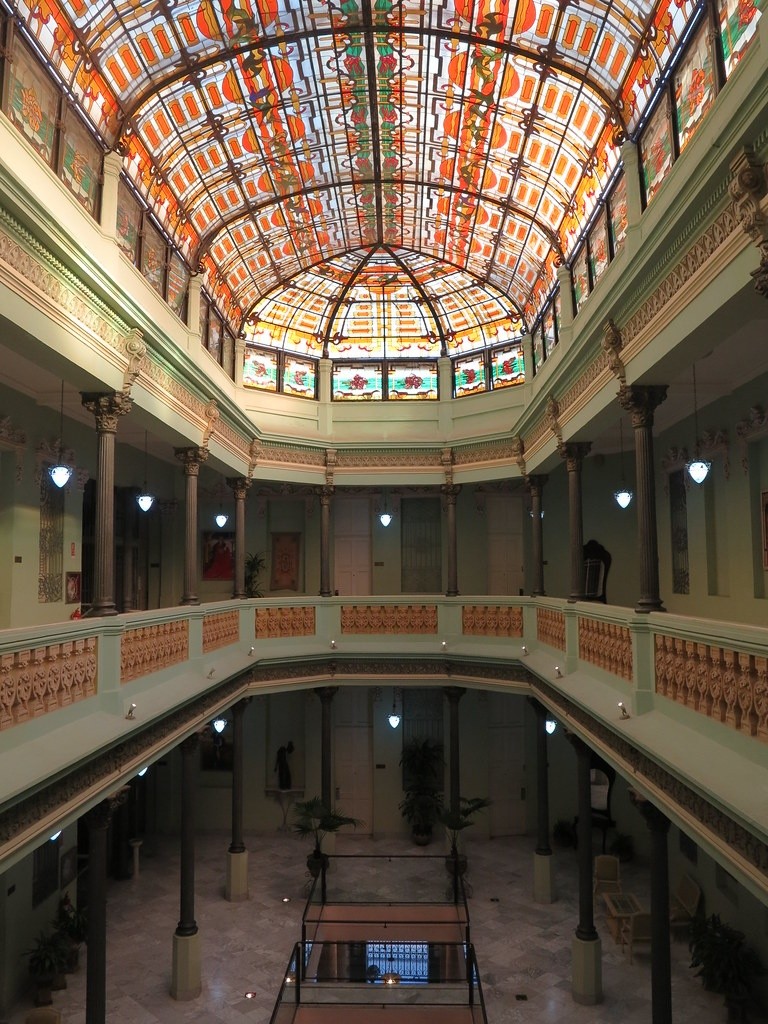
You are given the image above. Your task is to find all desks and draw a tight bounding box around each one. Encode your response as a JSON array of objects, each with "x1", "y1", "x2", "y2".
[
  {"x1": 601, "y1": 891, "x2": 642, "y2": 946},
  {"x1": 265, "y1": 784, "x2": 304, "y2": 831}
]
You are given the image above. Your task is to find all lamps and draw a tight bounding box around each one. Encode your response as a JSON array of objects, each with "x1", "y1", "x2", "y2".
[
  {"x1": 617, "y1": 701, "x2": 630, "y2": 720},
  {"x1": 248, "y1": 647, "x2": 255, "y2": 657},
  {"x1": 206, "y1": 668, "x2": 217, "y2": 679},
  {"x1": 554, "y1": 666, "x2": 563, "y2": 679},
  {"x1": 424, "y1": 794, "x2": 492, "y2": 875},
  {"x1": 47, "y1": 380, "x2": 74, "y2": 489},
  {"x1": 522, "y1": 647, "x2": 529, "y2": 656},
  {"x1": 381, "y1": 944, "x2": 399, "y2": 985},
  {"x1": 214, "y1": 715, "x2": 227, "y2": 733},
  {"x1": 387, "y1": 688, "x2": 401, "y2": 729},
  {"x1": 214, "y1": 485, "x2": 229, "y2": 528},
  {"x1": 125, "y1": 704, "x2": 137, "y2": 719},
  {"x1": 331, "y1": 640, "x2": 337, "y2": 650},
  {"x1": 377, "y1": 488, "x2": 393, "y2": 528},
  {"x1": 546, "y1": 714, "x2": 557, "y2": 736},
  {"x1": 683, "y1": 364, "x2": 711, "y2": 484},
  {"x1": 528, "y1": 505, "x2": 545, "y2": 519},
  {"x1": 136, "y1": 429, "x2": 156, "y2": 512},
  {"x1": 614, "y1": 417, "x2": 632, "y2": 509}
]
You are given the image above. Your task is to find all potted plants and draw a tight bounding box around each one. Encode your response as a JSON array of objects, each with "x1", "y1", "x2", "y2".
[
  {"x1": 19, "y1": 931, "x2": 67, "y2": 1008},
  {"x1": 290, "y1": 797, "x2": 368, "y2": 876},
  {"x1": 675, "y1": 914, "x2": 768, "y2": 1024},
  {"x1": 554, "y1": 818, "x2": 575, "y2": 847},
  {"x1": 47, "y1": 892, "x2": 88, "y2": 972},
  {"x1": 615, "y1": 830, "x2": 633, "y2": 861},
  {"x1": 398, "y1": 734, "x2": 447, "y2": 846}
]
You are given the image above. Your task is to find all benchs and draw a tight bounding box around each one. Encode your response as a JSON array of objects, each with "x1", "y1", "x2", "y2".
[{"x1": 669, "y1": 870, "x2": 701, "y2": 946}]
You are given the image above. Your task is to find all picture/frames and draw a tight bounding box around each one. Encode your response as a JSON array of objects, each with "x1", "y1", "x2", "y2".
[
  {"x1": 65, "y1": 571, "x2": 82, "y2": 603},
  {"x1": 200, "y1": 530, "x2": 236, "y2": 581},
  {"x1": 759, "y1": 488, "x2": 768, "y2": 571}
]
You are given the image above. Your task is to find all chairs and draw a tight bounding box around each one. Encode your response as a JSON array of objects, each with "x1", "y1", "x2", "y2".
[
  {"x1": 620, "y1": 911, "x2": 652, "y2": 964},
  {"x1": 594, "y1": 856, "x2": 622, "y2": 904}
]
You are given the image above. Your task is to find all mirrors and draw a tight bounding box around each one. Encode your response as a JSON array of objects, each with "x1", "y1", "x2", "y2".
[
  {"x1": 582, "y1": 539, "x2": 612, "y2": 604},
  {"x1": 571, "y1": 752, "x2": 615, "y2": 856}
]
